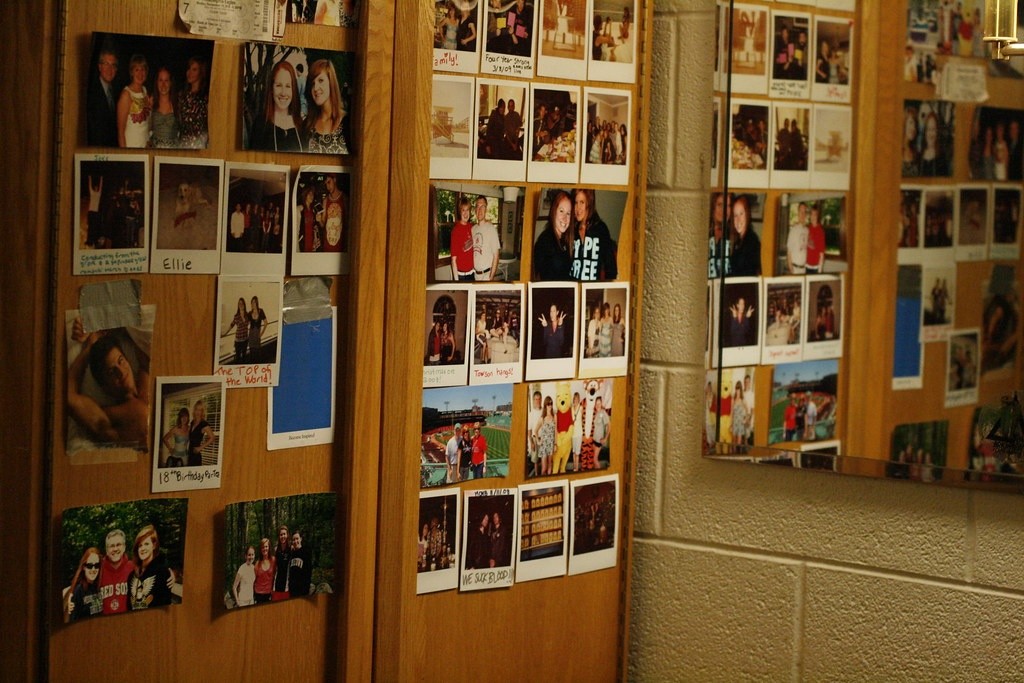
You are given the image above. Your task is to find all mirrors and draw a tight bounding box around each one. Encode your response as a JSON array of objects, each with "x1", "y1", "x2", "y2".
[{"x1": 700, "y1": 1, "x2": 1024, "y2": 498}]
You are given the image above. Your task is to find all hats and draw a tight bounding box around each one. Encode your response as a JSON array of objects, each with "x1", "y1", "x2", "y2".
[{"x1": 454, "y1": 423, "x2": 461, "y2": 430}]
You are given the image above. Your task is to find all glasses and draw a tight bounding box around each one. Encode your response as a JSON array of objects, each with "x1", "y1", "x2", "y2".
[
  {"x1": 99, "y1": 60, "x2": 117, "y2": 70},
  {"x1": 474, "y1": 428, "x2": 480, "y2": 431},
  {"x1": 547, "y1": 402, "x2": 553, "y2": 406},
  {"x1": 83, "y1": 563, "x2": 100, "y2": 569},
  {"x1": 462, "y1": 431, "x2": 469, "y2": 434},
  {"x1": 476, "y1": 205, "x2": 486, "y2": 208}
]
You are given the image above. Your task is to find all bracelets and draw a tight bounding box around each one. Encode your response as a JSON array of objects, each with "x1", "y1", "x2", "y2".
[{"x1": 169, "y1": 447, "x2": 171, "y2": 448}]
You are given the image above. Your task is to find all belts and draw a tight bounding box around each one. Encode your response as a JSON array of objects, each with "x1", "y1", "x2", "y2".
[
  {"x1": 458, "y1": 269, "x2": 474, "y2": 276},
  {"x1": 791, "y1": 262, "x2": 805, "y2": 269},
  {"x1": 807, "y1": 265, "x2": 818, "y2": 270},
  {"x1": 476, "y1": 268, "x2": 491, "y2": 274}
]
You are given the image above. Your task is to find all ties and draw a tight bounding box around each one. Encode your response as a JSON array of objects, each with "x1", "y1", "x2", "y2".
[{"x1": 107, "y1": 89, "x2": 112, "y2": 107}]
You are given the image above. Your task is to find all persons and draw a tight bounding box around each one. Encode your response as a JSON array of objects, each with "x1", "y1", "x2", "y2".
[
  {"x1": 288, "y1": 0, "x2": 360, "y2": 28},
  {"x1": 231, "y1": 203, "x2": 282, "y2": 253},
  {"x1": 223, "y1": 295, "x2": 267, "y2": 362},
  {"x1": 889, "y1": 0, "x2": 1024, "y2": 491},
  {"x1": 416, "y1": 0, "x2": 638, "y2": 578},
  {"x1": 163, "y1": 398, "x2": 215, "y2": 467},
  {"x1": 68, "y1": 316, "x2": 150, "y2": 441},
  {"x1": 295, "y1": 174, "x2": 350, "y2": 252},
  {"x1": 232, "y1": 525, "x2": 311, "y2": 607},
  {"x1": 63, "y1": 524, "x2": 176, "y2": 623},
  {"x1": 242, "y1": 54, "x2": 350, "y2": 155},
  {"x1": 90, "y1": 48, "x2": 207, "y2": 150},
  {"x1": 83, "y1": 173, "x2": 142, "y2": 249},
  {"x1": 707, "y1": 0, "x2": 853, "y2": 468}
]
